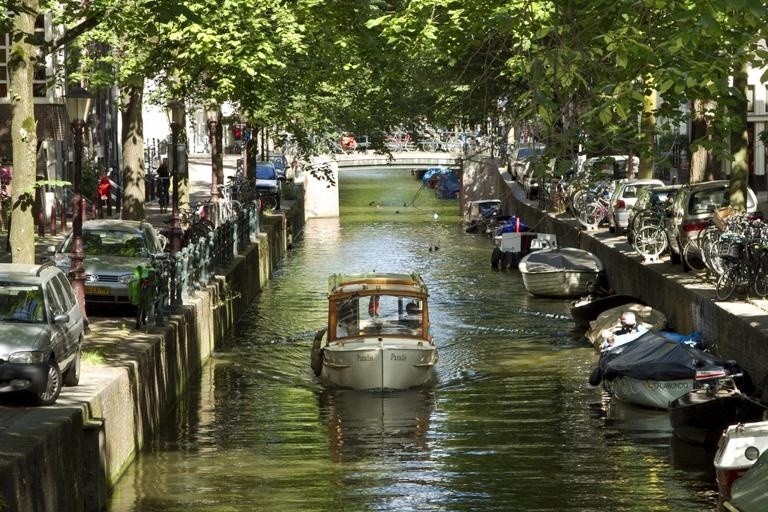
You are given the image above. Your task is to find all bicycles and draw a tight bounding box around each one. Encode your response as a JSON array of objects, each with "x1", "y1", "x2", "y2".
[
  {"x1": 158, "y1": 181, "x2": 278, "y2": 256},
  {"x1": 383, "y1": 122, "x2": 498, "y2": 157},
  {"x1": 565, "y1": 172, "x2": 768, "y2": 304}
]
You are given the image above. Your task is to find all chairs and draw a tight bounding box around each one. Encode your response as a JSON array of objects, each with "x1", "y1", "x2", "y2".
[
  {"x1": 692, "y1": 191, "x2": 730, "y2": 211},
  {"x1": 81, "y1": 233, "x2": 145, "y2": 256},
  {"x1": 9, "y1": 290, "x2": 38, "y2": 316}
]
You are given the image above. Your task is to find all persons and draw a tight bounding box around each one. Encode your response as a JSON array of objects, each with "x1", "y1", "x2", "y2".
[
  {"x1": 153, "y1": 162, "x2": 171, "y2": 210},
  {"x1": 435, "y1": 143, "x2": 445, "y2": 152},
  {"x1": 401, "y1": 304, "x2": 419, "y2": 327},
  {"x1": 410, "y1": 309, "x2": 435, "y2": 345},
  {"x1": 335, "y1": 312, "x2": 361, "y2": 338}
]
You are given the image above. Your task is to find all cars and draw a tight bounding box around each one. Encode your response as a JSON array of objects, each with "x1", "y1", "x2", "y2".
[
  {"x1": 507, "y1": 144, "x2": 556, "y2": 201},
  {"x1": 255, "y1": 152, "x2": 290, "y2": 200},
  {"x1": 49, "y1": 215, "x2": 166, "y2": 312},
  {"x1": 0, "y1": 259, "x2": 85, "y2": 406}
]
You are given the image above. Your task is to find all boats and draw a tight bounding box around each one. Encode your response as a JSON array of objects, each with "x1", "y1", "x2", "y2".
[
  {"x1": 517, "y1": 243, "x2": 603, "y2": 299},
  {"x1": 310, "y1": 268, "x2": 440, "y2": 392}
]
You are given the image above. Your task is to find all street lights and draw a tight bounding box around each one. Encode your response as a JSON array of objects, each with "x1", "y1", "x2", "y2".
[
  {"x1": 60, "y1": 79, "x2": 95, "y2": 337},
  {"x1": 203, "y1": 103, "x2": 221, "y2": 204},
  {"x1": 163, "y1": 99, "x2": 187, "y2": 259}
]
